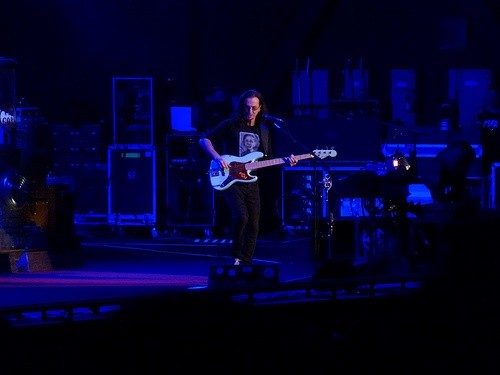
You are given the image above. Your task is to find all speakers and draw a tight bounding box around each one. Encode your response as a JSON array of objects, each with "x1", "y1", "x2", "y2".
[
  {"x1": 165, "y1": 133, "x2": 217, "y2": 228},
  {"x1": 210, "y1": 264, "x2": 279, "y2": 295}
]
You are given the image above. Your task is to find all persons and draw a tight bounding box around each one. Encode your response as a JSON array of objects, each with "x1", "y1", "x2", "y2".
[
  {"x1": 0, "y1": 122, "x2": 96, "y2": 261},
  {"x1": 199, "y1": 90, "x2": 298, "y2": 268}
]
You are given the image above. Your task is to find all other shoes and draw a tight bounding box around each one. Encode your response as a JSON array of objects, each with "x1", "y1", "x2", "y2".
[{"x1": 233, "y1": 257, "x2": 242, "y2": 265}]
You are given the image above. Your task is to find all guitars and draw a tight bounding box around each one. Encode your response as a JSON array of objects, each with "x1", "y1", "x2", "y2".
[{"x1": 207, "y1": 145, "x2": 337, "y2": 191}]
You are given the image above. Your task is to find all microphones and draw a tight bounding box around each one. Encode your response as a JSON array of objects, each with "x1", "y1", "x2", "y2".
[{"x1": 262, "y1": 115, "x2": 284, "y2": 122}]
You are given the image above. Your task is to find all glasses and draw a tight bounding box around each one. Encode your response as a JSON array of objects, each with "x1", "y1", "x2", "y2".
[{"x1": 244, "y1": 105, "x2": 260, "y2": 111}]
